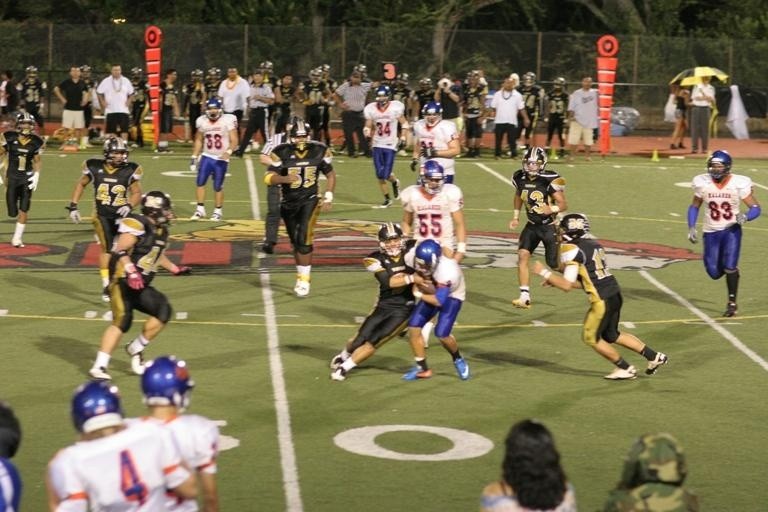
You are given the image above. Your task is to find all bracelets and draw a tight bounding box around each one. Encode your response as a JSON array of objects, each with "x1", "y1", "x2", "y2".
[
  {"x1": 513, "y1": 209, "x2": 520, "y2": 219},
  {"x1": 551, "y1": 206, "x2": 559, "y2": 211},
  {"x1": 540, "y1": 269, "x2": 552, "y2": 278}
]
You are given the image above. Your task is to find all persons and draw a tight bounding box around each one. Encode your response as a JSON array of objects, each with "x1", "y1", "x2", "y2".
[
  {"x1": 509, "y1": 145, "x2": 567, "y2": 310},
  {"x1": 664, "y1": 77, "x2": 715, "y2": 153},
  {"x1": 687, "y1": 150, "x2": 761, "y2": 316},
  {"x1": 140, "y1": 356, "x2": 220, "y2": 512},
  {"x1": 401, "y1": 160, "x2": 467, "y2": 347},
  {"x1": 66, "y1": 136, "x2": 143, "y2": 303},
  {"x1": 409, "y1": 102, "x2": 461, "y2": 184},
  {"x1": 255, "y1": 115, "x2": 336, "y2": 296},
  {"x1": 0, "y1": 404, "x2": 23, "y2": 512},
  {"x1": 0, "y1": 61, "x2": 599, "y2": 160},
  {"x1": 329, "y1": 222, "x2": 469, "y2": 379},
  {"x1": 480, "y1": 419, "x2": 578, "y2": 512},
  {"x1": 89, "y1": 191, "x2": 192, "y2": 379},
  {"x1": 531, "y1": 213, "x2": 669, "y2": 380},
  {"x1": 190, "y1": 98, "x2": 238, "y2": 221},
  {"x1": 604, "y1": 432, "x2": 699, "y2": 512},
  {"x1": 363, "y1": 86, "x2": 408, "y2": 207},
  {"x1": 44, "y1": 380, "x2": 200, "y2": 512},
  {"x1": 1, "y1": 112, "x2": 44, "y2": 246}
]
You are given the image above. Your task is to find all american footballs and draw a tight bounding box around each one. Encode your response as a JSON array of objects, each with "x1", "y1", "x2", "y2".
[{"x1": 417, "y1": 281, "x2": 436, "y2": 295}]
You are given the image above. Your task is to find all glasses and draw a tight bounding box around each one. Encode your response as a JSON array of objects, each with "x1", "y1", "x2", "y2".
[{"x1": 378, "y1": 224, "x2": 406, "y2": 257}]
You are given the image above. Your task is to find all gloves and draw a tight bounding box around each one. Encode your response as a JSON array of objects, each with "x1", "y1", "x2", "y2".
[
  {"x1": 70, "y1": 210, "x2": 81, "y2": 225},
  {"x1": 27, "y1": 170, "x2": 40, "y2": 191},
  {"x1": 125, "y1": 264, "x2": 146, "y2": 288},
  {"x1": 736, "y1": 213, "x2": 747, "y2": 226},
  {"x1": 115, "y1": 206, "x2": 130, "y2": 218},
  {"x1": 173, "y1": 266, "x2": 193, "y2": 275},
  {"x1": 688, "y1": 229, "x2": 699, "y2": 244}
]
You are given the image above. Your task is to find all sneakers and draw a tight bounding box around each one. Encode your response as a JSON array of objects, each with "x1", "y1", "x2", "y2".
[
  {"x1": 126, "y1": 341, "x2": 146, "y2": 375},
  {"x1": 380, "y1": 192, "x2": 391, "y2": 208},
  {"x1": 723, "y1": 303, "x2": 738, "y2": 317},
  {"x1": 512, "y1": 296, "x2": 531, "y2": 309},
  {"x1": 89, "y1": 365, "x2": 113, "y2": 380},
  {"x1": 392, "y1": 181, "x2": 399, "y2": 199},
  {"x1": 189, "y1": 207, "x2": 206, "y2": 222},
  {"x1": 260, "y1": 244, "x2": 274, "y2": 253},
  {"x1": 210, "y1": 206, "x2": 224, "y2": 221},
  {"x1": 295, "y1": 281, "x2": 311, "y2": 296},
  {"x1": 12, "y1": 237, "x2": 24, "y2": 247}
]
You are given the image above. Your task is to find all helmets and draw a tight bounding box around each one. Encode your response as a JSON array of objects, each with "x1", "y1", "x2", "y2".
[
  {"x1": 558, "y1": 213, "x2": 590, "y2": 242},
  {"x1": 291, "y1": 118, "x2": 314, "y2": 154},
  {"x1": 418, "y1": 161, "x2": 447, "y2": 195},
  {"x1": 415, "y1": 240, "x2": 443, "y2": 273},
  {"x1": 141, "y1": 356, "x2": 194, "y2": 411},
  {"x1": 707, "y1": 149, "x2": 732, "y2": 178},
  {"x1": 523, "y1": 145, "x2": 548, "y2": 173},
  {"x1": 70, "y1": 383, "x2": 124, "y2": 433}
]
